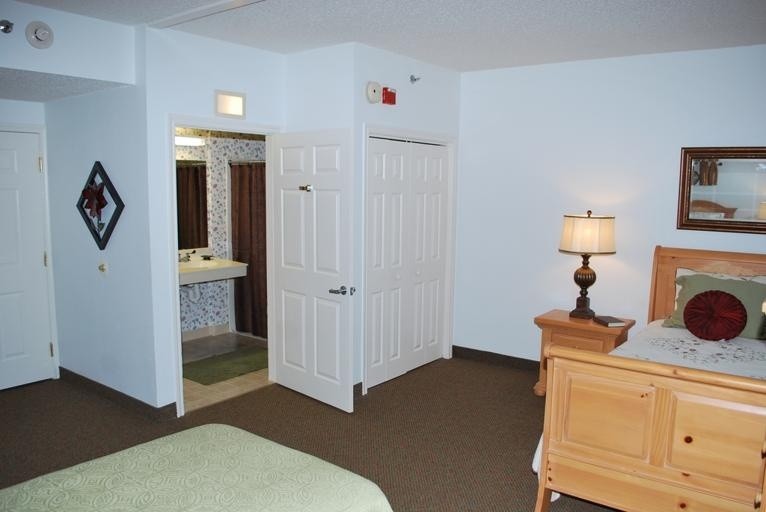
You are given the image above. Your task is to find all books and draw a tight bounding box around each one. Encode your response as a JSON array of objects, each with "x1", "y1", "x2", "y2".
[{"x1": 593, "y1": 315, "x2": 627, "y2": 328}]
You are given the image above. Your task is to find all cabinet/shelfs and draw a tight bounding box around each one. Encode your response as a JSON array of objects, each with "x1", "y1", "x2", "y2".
[{"x1": 364, "y1": 120, "x2": 450, "y2": 390}]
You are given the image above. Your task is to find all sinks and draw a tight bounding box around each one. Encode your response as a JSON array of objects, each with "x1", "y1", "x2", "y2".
[{"x1": 179, "y1": 259, "x2": 248, "y2": 286}]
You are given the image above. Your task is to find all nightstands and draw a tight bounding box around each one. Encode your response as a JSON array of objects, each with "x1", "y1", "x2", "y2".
[{"x1": 532, "y1": 309, "x2": 635, "y2": 397}]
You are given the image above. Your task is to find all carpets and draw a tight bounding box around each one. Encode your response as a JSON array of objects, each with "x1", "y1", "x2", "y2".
[{"x1": 183, "y1": 344, "x2": 268, "y2": 386}]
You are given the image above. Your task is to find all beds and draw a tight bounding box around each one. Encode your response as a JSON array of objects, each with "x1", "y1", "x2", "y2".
[
  {"x1": 530, "y1": 245, "x2": 766, "y2": 512},
  {"x1": 0, "y1": 423, "x2": 394, "y2": 512}
]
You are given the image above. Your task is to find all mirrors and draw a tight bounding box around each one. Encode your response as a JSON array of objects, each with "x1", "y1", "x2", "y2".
[{"x1": 176, "y1": 160, "x2": 208, "y2": 250}]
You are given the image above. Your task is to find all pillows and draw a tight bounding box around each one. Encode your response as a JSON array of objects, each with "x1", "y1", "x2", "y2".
[{"x1": 668, "y1": 266, "x2": 765, "y2": 344}]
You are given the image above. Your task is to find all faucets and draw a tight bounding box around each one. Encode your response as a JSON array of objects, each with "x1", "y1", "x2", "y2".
[{"x1": 179, "y1": 250, "x2": 196, "y2": 262}]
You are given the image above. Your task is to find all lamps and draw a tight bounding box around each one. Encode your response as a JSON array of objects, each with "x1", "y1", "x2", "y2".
[{"x1": 558, "y1": 208, "x2": 617, "y2": 320}]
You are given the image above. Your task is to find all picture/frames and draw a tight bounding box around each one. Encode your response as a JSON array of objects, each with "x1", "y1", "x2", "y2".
[
  {"x1": 676, "y1": 146, "x2": 766, "y2": 234},
  {"x1": 75, "y1": 162, "x2": 124, "y2": 250}
]
